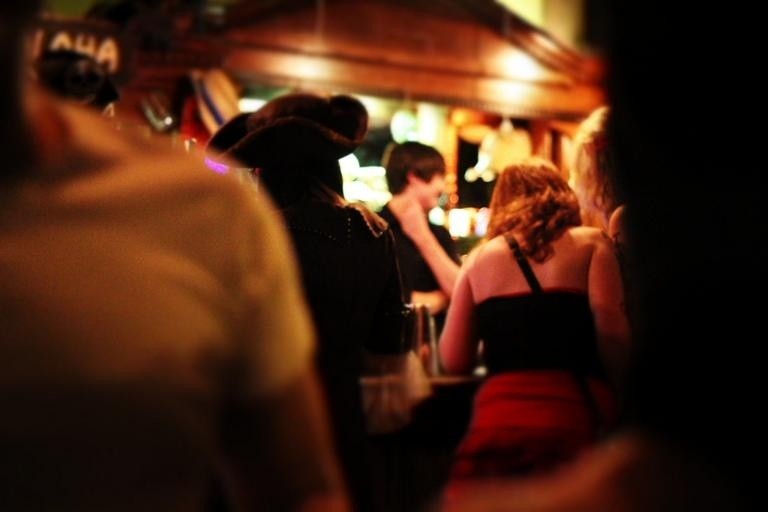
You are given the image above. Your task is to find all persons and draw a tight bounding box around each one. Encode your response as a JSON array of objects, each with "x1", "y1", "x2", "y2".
[
  {"x1": 434, "y1": 158, "x2": 626, "y2": 510},
  {"x1": 0, "y1": 0, "x2": 354, "y2": 510},
  {"x1": 373, "y1": 139, "x2": 464, "y2": 354},
  {"x1": 199, "y1": 90, "x2": 412, "y2": 467},
  {"x1": 570, "y1": 102, "x2": 617, "y2": 225}
]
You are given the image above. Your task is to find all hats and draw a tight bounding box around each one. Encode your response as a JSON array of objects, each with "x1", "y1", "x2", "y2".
[{"x1": 204, "y1": 91, "x2": 371, "y2": 171}]
